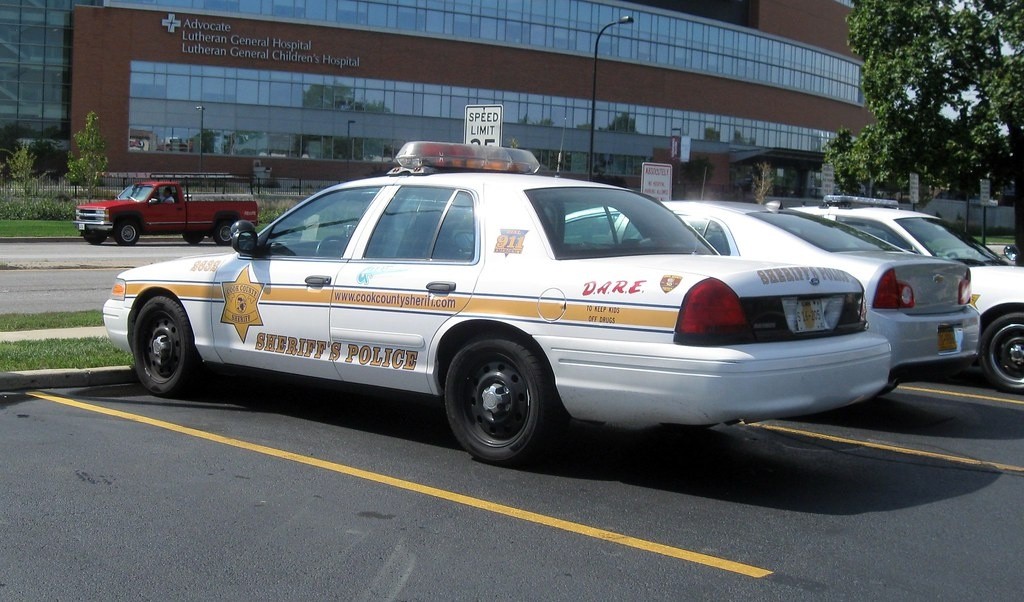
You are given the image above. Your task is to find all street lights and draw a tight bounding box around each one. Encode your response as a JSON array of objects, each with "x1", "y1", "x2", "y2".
[
  {"x1": 195, "y1": 105, "x2": 205, "y2": 173},
  {"x1": 586, "y1": 15, "x2": 634, "y2": 183}
]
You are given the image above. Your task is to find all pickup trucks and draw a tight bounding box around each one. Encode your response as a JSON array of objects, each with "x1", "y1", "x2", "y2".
[{"x1": 73, "y1": 180, "x2": 259, "y2": 247}]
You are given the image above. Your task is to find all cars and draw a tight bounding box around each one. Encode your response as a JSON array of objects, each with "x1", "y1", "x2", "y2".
[
  {"x1": 100, "y1": 142, "x2": 890, "y2": 468},
  {"x1": 786, "y1": 193, "x2": 1024, "y2": 396},
  {"x1": 563, "y1": 201, "x2": 981, "y2": 419}
]
